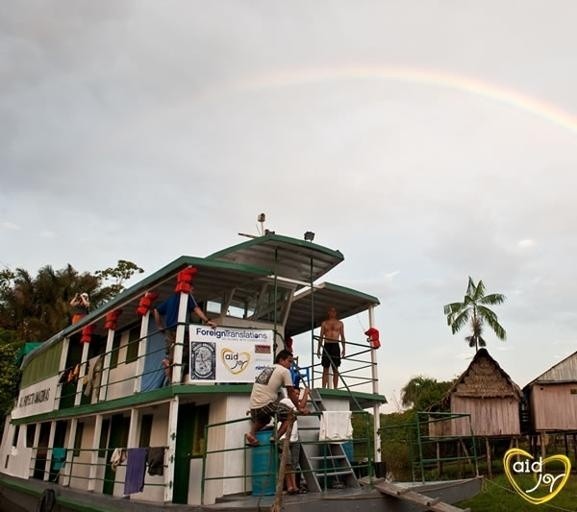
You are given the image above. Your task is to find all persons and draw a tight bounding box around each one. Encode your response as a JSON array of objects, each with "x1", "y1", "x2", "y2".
[
  {"x1": 278, "y1": 387, "x2": 311, "y2": 495},
  {"x1": 67, "y1": 364, "x2": 79, "y2": 384},
  {"x1": 246, "y1": 350, "x2": 311, "y2": 443},
  {"x1": 154, "y1": 283, "x2": 217, "y2": 368},
  {"x1": 70, "y1": 293, "x2": 90, "y2": 324},
  {"x1": 317, "y1": 307, "x2": 346, "y2": 389}
]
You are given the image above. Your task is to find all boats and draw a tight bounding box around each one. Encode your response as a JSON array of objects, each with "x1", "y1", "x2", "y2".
[{"x1": 0, "y1": 212, "x2": 483, "y2": 512}]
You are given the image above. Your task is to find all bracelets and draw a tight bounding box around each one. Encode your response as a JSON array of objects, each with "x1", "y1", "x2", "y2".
[{"x1": 206, "y1": 320, "x2": 210, "y2": 324}]
